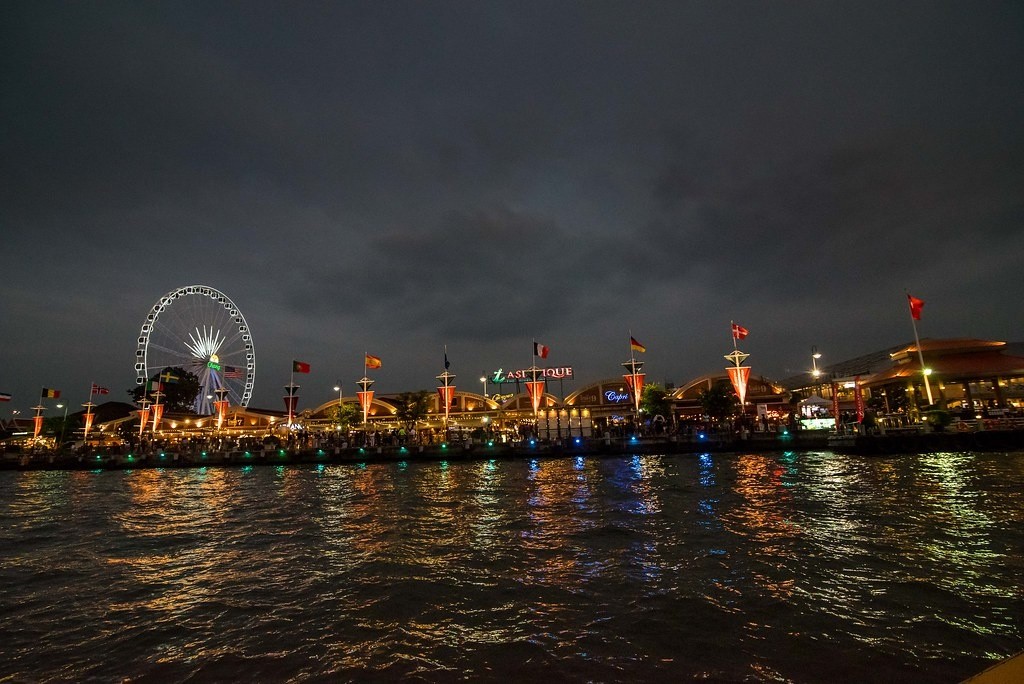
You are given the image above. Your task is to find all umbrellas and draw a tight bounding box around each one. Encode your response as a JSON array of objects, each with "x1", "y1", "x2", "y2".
[{"x1": 797, "y1": 395, "x2": 833, "y2": 407}]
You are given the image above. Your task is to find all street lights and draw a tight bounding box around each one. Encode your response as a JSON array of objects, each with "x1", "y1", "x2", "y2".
[
  {"x1": 55, "y1": 397, "x2": 71, "y2": 447},
  {"x1": 810, "y1": 345, "x2": 824, "y2": 396},
  {"x1": 478, "y1": 369, "x2": 488, "y2": 411},
  {"x1": 333, "y1": 379, "x2": 343, "y2": 425}
]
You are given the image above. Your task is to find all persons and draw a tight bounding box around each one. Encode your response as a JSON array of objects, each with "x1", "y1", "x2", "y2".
[
  {"x1": 596, "y1": 408, "x2": 832, "y2": 440},
  {"x1": 0, "y1": 435, "x2": 264, "y2": 457},
  {"x1": 287, "y1": 423, "x2": 542, "y2": 450},
  {"x1": 839, "y1": 401, "x2": 1023, "y2": 431}
]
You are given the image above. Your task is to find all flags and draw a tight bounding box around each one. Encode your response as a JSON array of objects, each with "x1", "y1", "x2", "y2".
[
  {"x1": 145, "y1": 381, "x2": 164, "y2": 391},
  {"x1": 630, "y1": 335, "x2": 646, "y2": 353},
  {"x1": 365, "y1": 354, "x2": 382, "y2": 370},
  {"x1": 91, "y1": 383, "x2": 110, "y2": 395},
  {"x1": 731, "y1": 323, "x2": 751, "y2": 342},
  {"x1": 533, "y1": 341, "x2": 550, "y2": 361},
  {"x1": 908, "y1": 293, "x2": 925, "y2": 321},
  {"x1": 0, "y1": 392, "x2": 13, "y2": 403},
  {"x1": 291, "y1": 360, "x2": 310, "y2": 374},
  {"x1": 223, "y1": 365, "x2": 243, "y2": 380},
  {"x1": 41, "y1": 388, "x2": 61, "y2": 399},
  {"x1": 159, "y1": 369, "x2": 181, "y2": 384},
  {"x1": 444, "y1": 351, "x2": 450, "y2": 372}
]
